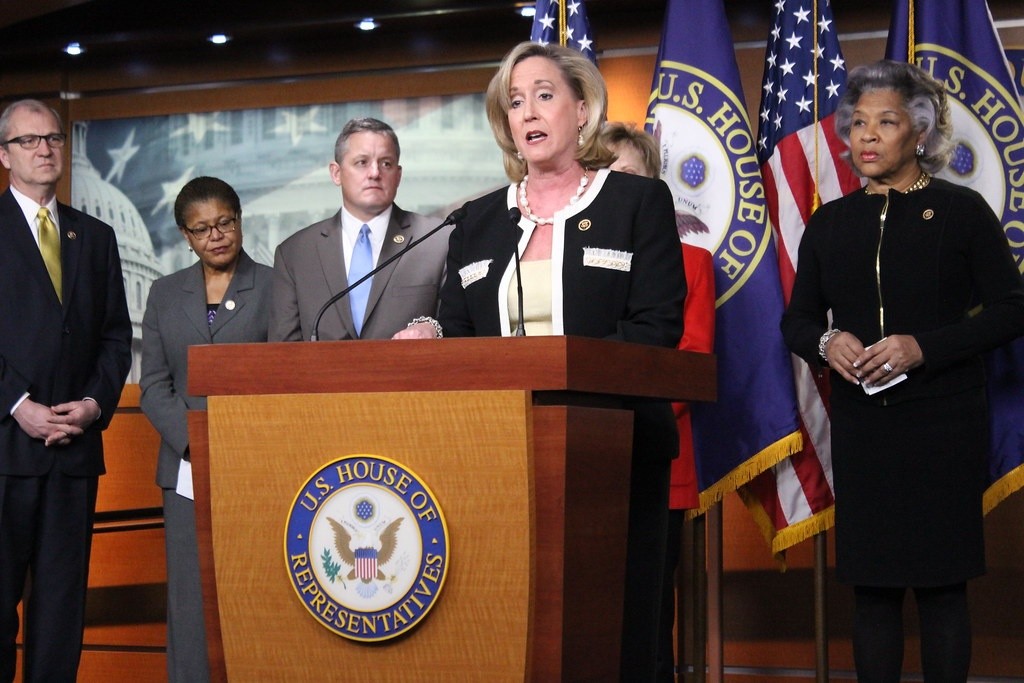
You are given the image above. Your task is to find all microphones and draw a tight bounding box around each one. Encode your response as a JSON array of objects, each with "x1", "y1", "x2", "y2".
[
  {"x1": 309, "y1": 208, "x2": 467, "y2": 342},
  {"x1": 508, "y1": 207, "x2": 526, "y2": 335}
]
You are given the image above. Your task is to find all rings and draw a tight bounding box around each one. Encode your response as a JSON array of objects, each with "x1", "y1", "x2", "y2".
[{"x1": 884, "y1": 363, "x2": 893, "y2": 372}]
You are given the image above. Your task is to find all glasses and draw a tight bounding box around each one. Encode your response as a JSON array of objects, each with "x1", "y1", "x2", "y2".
[
  {"x1": 0, "y1": 133, "x2": 67, "y2": 149},
  {"x1": 182, "y1": 213, "x2": 240, "y2": 241}
]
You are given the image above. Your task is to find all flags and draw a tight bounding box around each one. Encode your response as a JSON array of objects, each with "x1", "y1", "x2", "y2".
[
  {"x1": 530, "y1": 0, "x2": 598, "y2": 68},
  {"x1": 642, "y1": 0, "x2": 1024, "y2": 562}
]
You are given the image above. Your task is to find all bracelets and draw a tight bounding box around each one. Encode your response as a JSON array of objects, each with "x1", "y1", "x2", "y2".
[{"x1": 819, "y1": 328, "x2": 841, "y2": 361}]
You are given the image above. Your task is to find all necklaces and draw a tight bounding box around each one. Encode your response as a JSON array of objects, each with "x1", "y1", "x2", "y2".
[
  {"x1": 518, "y1": 162, "x2": 591, "y2": 225},
  {"x1": 865, "y1": 171, "x2": 931, "y2": 198}
]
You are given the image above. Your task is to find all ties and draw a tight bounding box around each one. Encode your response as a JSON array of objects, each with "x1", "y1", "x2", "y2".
[
  {"x1": 37, "y1": 207, "x2": 63, "y2": 304},
  {"x1": 348, "y1": 224, "x2": 373, "y2": 338}
]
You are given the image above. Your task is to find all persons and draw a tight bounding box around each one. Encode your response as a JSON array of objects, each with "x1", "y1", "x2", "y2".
[
  {"x1": 780, "y1": 61, "x2": 1024, "y2": 683},
  {"x1": 599, "y1": 121, "x2": 715, "y2": 683},
  {"x1": 139, "y1": 176, "x2": 273, "y2": 683},
  {"x1": 391, "y1": 41, "x2": 688, "y2": 683},
  {"x1": 267, "y1": 117, "x2": 455, "y2": 343},
  {"x1": 0, "y1": 98, "x2": 133, "y2": 683}
]
